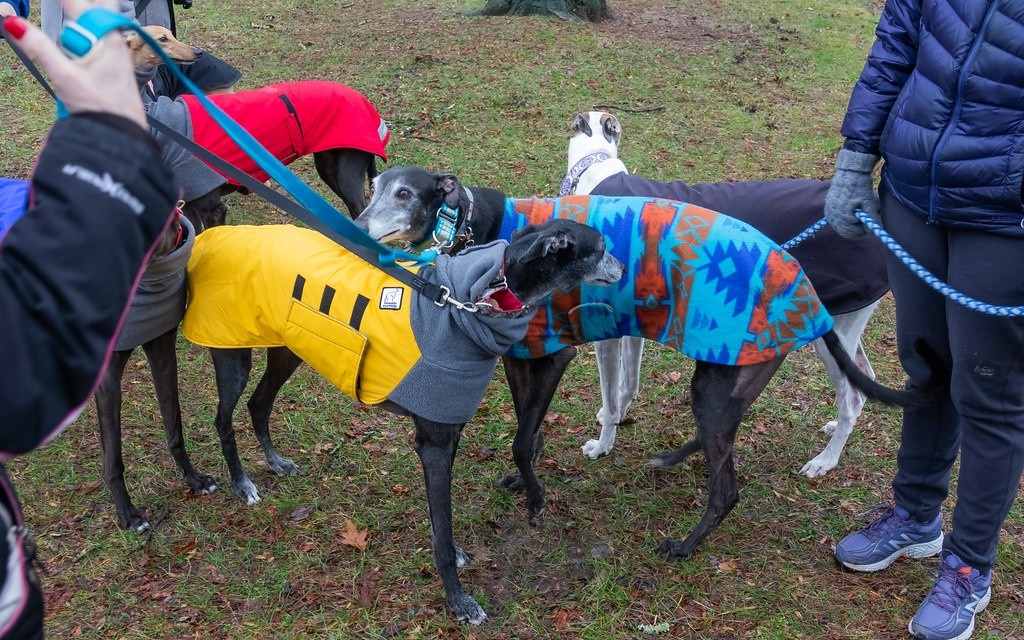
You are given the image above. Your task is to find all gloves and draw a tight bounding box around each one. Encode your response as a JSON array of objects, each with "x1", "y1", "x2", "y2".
[{"x1": 823, "y1": 149, "x2": 881, "y2": 241}]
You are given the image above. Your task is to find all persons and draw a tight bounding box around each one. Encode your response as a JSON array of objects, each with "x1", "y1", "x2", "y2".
[
  {"x1": 823, "y1": 0, "x2": 1024, "y2": 640},
  {"x1": 0, "y1": 0, "x2": 193, "y2": 640}
]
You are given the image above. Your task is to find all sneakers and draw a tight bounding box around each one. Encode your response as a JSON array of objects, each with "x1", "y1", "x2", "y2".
[
  {"x1": 908, "y1": 549, "x2": 992, "y2": 640},
  {"x1": 833, "y1": 502, "x2": 946, "y2": 572}
]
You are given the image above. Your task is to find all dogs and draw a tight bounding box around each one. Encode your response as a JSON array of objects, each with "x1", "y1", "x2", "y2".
[{"x1": 0, "y1": 24, "x2": 955, "y2": 628}]
